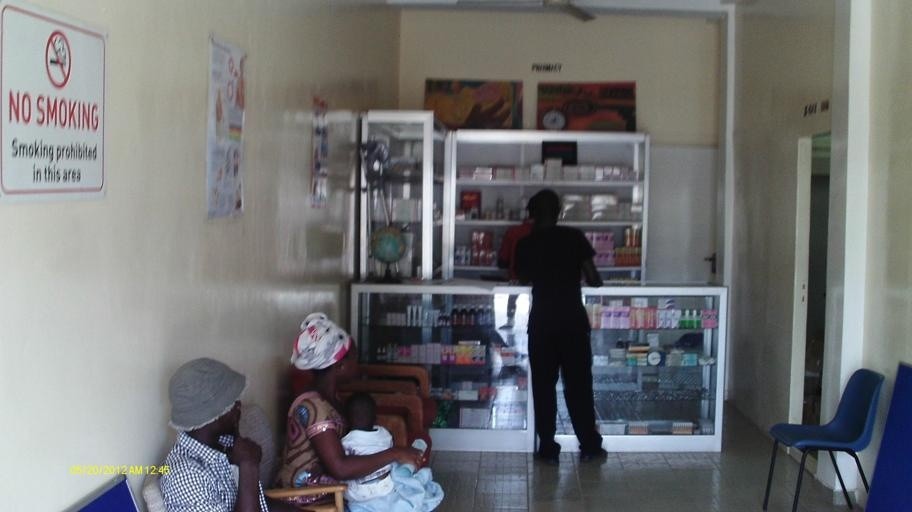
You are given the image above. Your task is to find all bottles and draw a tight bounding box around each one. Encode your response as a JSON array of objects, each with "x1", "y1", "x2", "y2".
[{"x1": 451, "y1": 304, "x2": 493, "y2": 326}]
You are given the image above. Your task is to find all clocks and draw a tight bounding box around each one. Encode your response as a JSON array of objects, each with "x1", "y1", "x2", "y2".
[{"x1": 541, "y1": 109, "x2": 568, "y2": 130}]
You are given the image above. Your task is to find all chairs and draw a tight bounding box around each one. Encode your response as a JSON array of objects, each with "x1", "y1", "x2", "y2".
[
  {"x1": 763, "y1": 368, "x2": 885, "y2": 512},
  {"x1": 64, "y1": 361, "x2": 437, "y2": 511}
]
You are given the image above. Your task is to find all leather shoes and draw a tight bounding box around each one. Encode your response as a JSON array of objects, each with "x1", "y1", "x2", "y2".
[{"x1": 532, "y1": 448, "x2": 607, "y2": 463}]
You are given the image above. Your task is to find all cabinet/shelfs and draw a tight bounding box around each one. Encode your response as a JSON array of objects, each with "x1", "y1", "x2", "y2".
[
  {"x1": 349, "y1": 279, "x2": 729, "y2": 455},
  {"x1": 441, "y1": 128, "x2": 650, "y2": 390},
  {"x1": 358, "y1": 109, "x2": 445, "y2": 388}
]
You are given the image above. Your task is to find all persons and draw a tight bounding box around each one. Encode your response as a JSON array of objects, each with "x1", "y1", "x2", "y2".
[
  {"x1": 305, "y1": 388, "x2": 395, "y2": 501},
  {"x1": 272, "y1": 311, "x2": 431, "y2": 511},
  {"x1": 496, "y1": 196, "x2": 535, "y2": 279},
  {"x1": 156, "y1": 356, "x2": 270, "y2": 511},
  {"x1": 510, "y1": 188, "x2": 608, "y2": 466}
]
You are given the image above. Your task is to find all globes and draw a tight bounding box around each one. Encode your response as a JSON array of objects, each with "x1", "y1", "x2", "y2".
[{"x1": 371, "y1": 226, "x2": 406, "y2": 283}]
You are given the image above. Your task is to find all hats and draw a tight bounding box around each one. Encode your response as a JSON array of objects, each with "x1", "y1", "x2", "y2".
[{"x1": 166, "y1": 357, "x2": 248, "y2": 435}]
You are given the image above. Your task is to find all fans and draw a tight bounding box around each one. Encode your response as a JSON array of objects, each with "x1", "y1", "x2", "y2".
[{"x1": 542, "y1": 0, "x2": 595, "y2": 22}]
